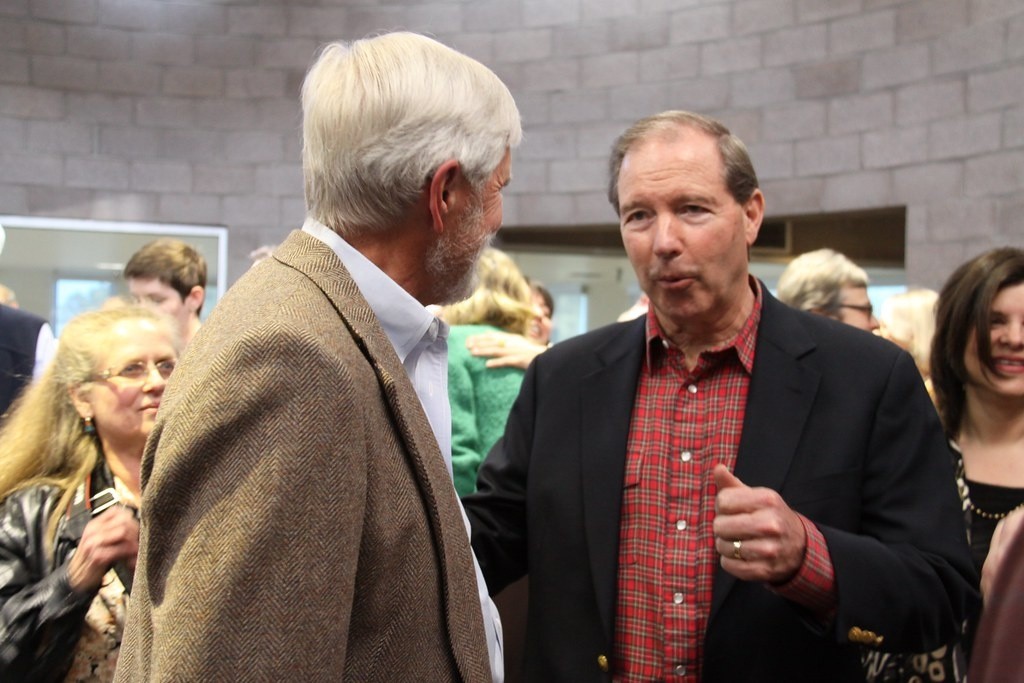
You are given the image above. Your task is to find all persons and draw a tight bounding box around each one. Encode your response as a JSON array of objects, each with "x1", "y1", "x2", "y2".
[
  {"x1": 860, "y1": 248, "x2": 1024, "y2": 683},
  {"x1": 113, "y1": 30, "x2": 521, "y2": 683},
  {"x1": 460, "y1": 110, "x2": 985, "y2": 683},
  {"x1": 0, "y1": 230, "x2": 56, "y2": 430},
  {"x1": 425, "y1": 246, "x2": 556, "y2": 498},
  {"x1": 777, "y1": 251, "x2": 880, "y2": 334},
  {"x1": 120, "y1": 238, "x2": 208, "y2": 349},
  {"x1": 0, "y1": 309, "x2": 182, "y2": 683}
]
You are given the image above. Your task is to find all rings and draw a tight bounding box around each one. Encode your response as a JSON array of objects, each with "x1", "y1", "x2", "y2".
[{"x1": 733, "y1": 540, "x2": 742, "y2": 559}]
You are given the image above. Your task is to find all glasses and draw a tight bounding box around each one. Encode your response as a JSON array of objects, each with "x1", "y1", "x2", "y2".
[
  {"x1": 829, "y1": 305, "x2": 872, "y2": 316},
  {"x1": 83, "y1": 360, "x2": 174, "y2": 383}
]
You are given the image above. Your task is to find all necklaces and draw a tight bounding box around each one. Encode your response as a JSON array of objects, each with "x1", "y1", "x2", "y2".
[{"x1": 955, "y1": 437, "x2": 1024, "y2": 518}]
